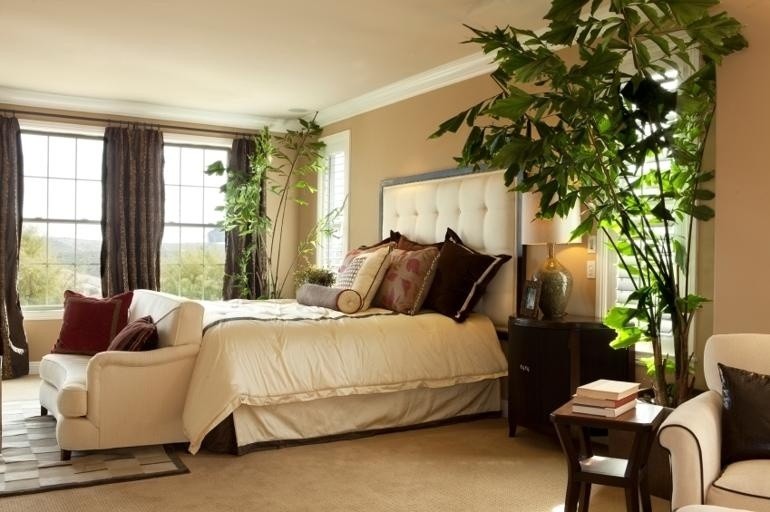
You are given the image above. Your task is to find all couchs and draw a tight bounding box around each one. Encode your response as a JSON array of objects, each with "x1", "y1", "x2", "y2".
[
  {"x1": 36, "y1": 288, "x2": 205, "y2": 460},
  {"x1": 654, "y1": 333, "x2": 770, "y2": 512}
]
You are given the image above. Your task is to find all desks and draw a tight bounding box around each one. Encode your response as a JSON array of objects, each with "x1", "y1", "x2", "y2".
[{"x1": 549, "y1": 396, "x2": 665, "y2": 512}]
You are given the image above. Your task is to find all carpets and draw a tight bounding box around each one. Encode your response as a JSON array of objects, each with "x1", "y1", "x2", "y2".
[{"x1": 0, "y1": 399, "x2": 191, "y2": 496}]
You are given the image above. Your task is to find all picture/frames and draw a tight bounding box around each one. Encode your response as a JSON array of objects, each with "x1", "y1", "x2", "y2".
[{"x1": 521, "y1": 278, "x2": 544, "y2": 321}]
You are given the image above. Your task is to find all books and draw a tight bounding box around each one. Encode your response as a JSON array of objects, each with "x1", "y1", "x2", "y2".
[{"x1": 571, "y1": 378, "x2": 642, "y2": 418}]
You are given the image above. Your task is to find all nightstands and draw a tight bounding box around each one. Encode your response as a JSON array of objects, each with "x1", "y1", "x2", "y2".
[{"x1": 508, "y1": 315, "x2": 635, "y2": 448}]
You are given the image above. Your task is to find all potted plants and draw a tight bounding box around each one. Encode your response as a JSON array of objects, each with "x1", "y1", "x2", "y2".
[{"x1": 429, "y1": 0, "x2": 750, "y2": 503}]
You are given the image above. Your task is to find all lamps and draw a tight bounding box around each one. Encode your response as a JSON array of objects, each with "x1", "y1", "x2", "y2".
[{"x1": 520, "y1": 164, "x2": 584, "y2": 318}]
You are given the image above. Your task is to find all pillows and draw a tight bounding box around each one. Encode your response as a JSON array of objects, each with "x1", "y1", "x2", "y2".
[
  {"x1": 106, "y1": 314, "x2": 159, "y2": 351},
  {"x1": 50, "y1": 290, "x2": 134, "y2": 356},
  {"x1": 295, "y1": 227, "x2": 513, "y2": 324},
  {"x1": 719, "y1": 364, "x2": 770, "y2": 477}
]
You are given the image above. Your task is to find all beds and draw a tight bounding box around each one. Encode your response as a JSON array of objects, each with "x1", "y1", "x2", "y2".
[{"x1": 178, "y1": 161, "x2": 524, "y2": 457}]
